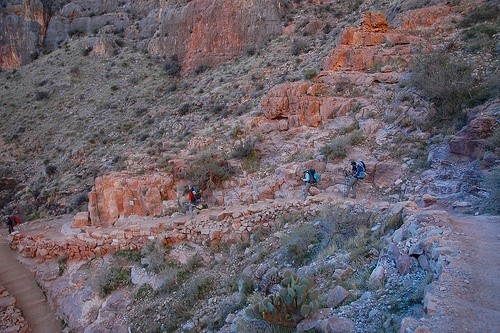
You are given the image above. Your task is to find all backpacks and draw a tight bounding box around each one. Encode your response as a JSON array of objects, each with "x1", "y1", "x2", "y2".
[
  {"x1": 309, "y1": 168, "x2": 318, "y2": 184},
  {"x1": 357, "y1": 161, "x2": 367, "y2": 179},
  {"x1": 12, "y1": 219, "x2": 17, "y2": 226},
  {"x1": 194, "y1": 188, "x2": 200, "y2": 205}
]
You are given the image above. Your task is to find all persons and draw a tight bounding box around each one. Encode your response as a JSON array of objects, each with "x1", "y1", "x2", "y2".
[
  {"x1": 6, "y1": 216, "x2": 17, "y2": 234},
  {"x1": 186, "y1": 185, "x2": 201, "y2": 219},
  {"x1": 300, "y1": 163, "x2": 320, "y2": 202},
  {"x1": 342, "y1": 157, "x2": 367, "y2": 198}
]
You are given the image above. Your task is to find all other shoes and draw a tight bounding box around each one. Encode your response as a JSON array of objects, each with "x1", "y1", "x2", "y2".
[
  {"x1": 344, "y1": 194, "x2": 348, "y2": 197},
  {"x1": 350, "y1": 196, "x2": 356, "y2": 198}
]
